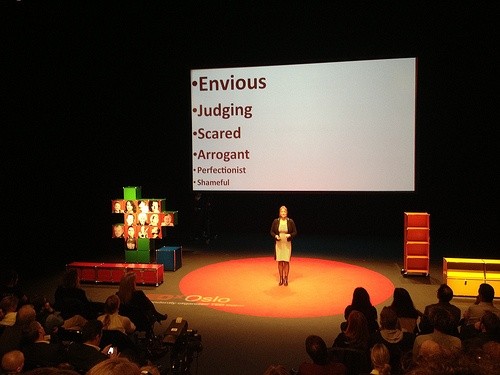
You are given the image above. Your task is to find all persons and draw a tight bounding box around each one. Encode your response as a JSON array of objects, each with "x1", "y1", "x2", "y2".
[
  {"x1": 302, "y1": 284, "x2": 500, "y2": 375},
  {"x1": 0, "y1": 269, "x2": 168, "y2": 375},
  {"x1": 113, "y1": 200, "x2": 173, "y2": 250},
  {"x1": 270, "y1": 205, "x2": 296, "y2": 286}
]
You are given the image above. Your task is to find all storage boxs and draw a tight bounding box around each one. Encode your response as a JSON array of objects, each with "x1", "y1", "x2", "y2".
[
  {"x1": 69, "y1": 261, "x2": 164, "y2": 286},
  {"x1": 156, "y1": 246, "x2": 182, "y2": 272}
]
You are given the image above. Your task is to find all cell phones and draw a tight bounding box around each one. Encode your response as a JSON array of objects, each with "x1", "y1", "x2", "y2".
[{"x1": 108, "y1": 346, "x2": 113, "y2": 355}]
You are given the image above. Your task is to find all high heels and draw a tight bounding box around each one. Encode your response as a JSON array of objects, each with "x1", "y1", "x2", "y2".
[{"x1": 157, "y1": 312, "x2": 168, "y2": 325}]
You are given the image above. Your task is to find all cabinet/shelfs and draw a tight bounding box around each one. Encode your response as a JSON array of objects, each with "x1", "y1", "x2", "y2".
[
  {"x1": 401, "y1": 212, "x2": 431, "y2": 276},
  {"x1": 443, "y1": 257, "x2": 500, "y2": 298}
]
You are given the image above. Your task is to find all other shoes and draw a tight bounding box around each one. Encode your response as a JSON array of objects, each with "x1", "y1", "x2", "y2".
[
  {"x1": 283, "y1": 281, "x2": 288, "y2": 286},
  {"x1": 279, "y1": 281, "x2": 283, "y2": 286}
]
